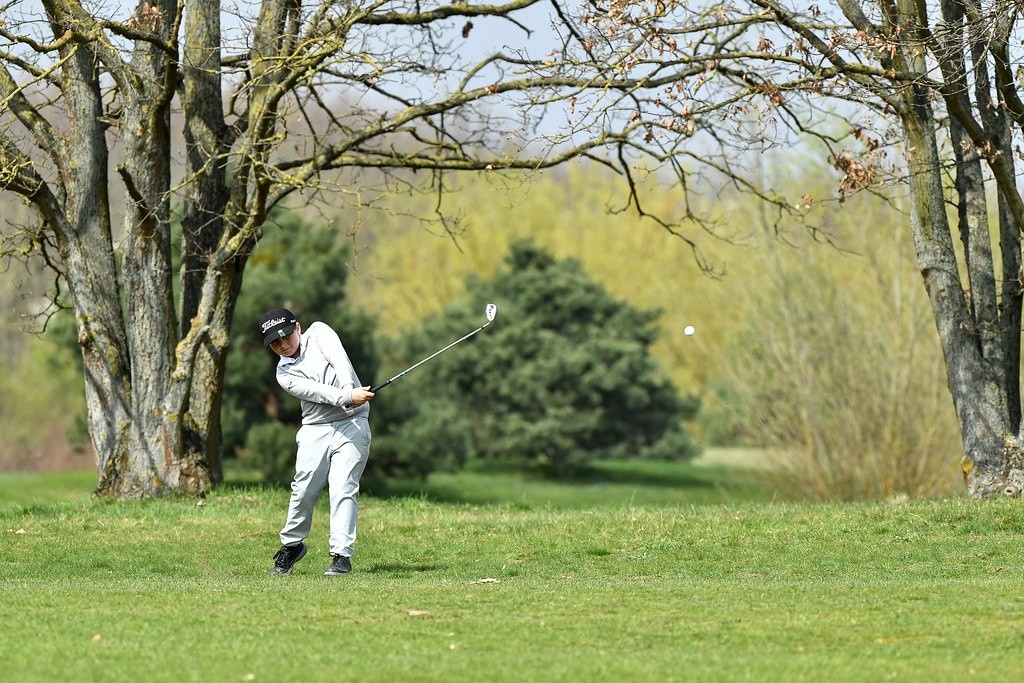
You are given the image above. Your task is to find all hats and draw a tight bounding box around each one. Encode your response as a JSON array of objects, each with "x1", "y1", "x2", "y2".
[{"x1": 258, "y1": 307, "x2": 297, "y2": 349}]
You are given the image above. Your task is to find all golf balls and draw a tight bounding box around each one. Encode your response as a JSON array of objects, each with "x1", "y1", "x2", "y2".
[{"x1": 684, "y1": 326, "x2": 695, "y2": 336}]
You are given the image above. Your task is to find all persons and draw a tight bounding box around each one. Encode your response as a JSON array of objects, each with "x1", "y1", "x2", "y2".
[{"x1": 260, "y1": 309, "x2": 374, "y2": 575}]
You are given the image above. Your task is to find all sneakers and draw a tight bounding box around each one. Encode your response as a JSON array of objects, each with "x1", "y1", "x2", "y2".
[
  {"x1": 271, "y1": 541, "x2": 307, "y2": 578},
  {"x1": 324, "y1": 551, "x2": 352, "y2": 576}
]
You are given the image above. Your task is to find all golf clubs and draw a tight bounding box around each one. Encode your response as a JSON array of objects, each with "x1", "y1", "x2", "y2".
[{"x1": 345, "y1": 303, "x2": 498, "y2": 409}]
